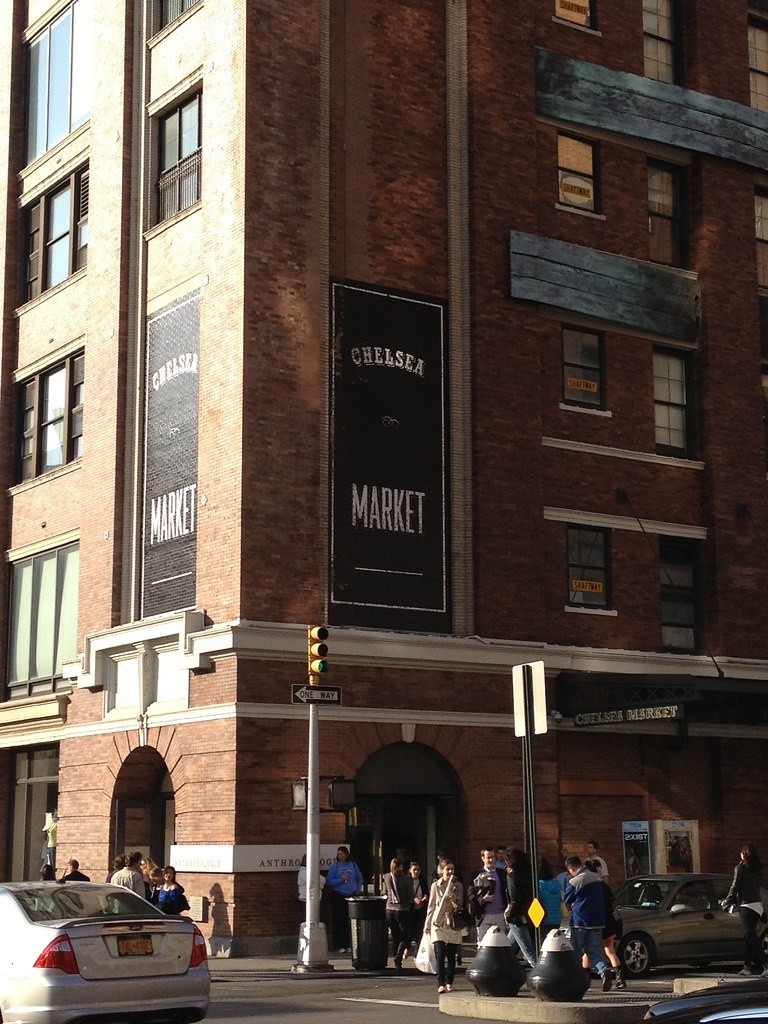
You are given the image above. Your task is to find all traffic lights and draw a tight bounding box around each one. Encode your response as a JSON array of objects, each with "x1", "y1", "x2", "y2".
[
  {"x1": 308, "y1": 624, "x2": 330, "y2": 676},
  {"x1": 291, "y1": 780, "x2": 309, "y2": 811},
  {"x1": 329, "y1": 779, "x2": 358, "y2": 808}
]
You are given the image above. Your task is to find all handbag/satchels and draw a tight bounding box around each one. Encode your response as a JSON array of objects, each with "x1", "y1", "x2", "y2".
[
  {"x1": 450, "y1": 909, "x2": 468, "y2": 930},
  {"x1": 413, "y1": 933, "x2": 438, "y2": 976}
]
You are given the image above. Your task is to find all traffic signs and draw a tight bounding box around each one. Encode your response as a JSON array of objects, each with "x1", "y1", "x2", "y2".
[{"x1": 291, "y1": 684, "x2": 342, "y2": 707}]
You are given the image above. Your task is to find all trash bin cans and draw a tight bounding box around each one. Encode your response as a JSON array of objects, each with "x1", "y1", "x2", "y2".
[{"x1": 345, "y1": 896, "x2": 388, "y2": 970}]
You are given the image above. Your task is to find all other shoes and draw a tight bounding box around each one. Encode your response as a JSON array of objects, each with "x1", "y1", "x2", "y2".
[
  {"x1": 438, "y1": 986, "x2": 445, "y2": 993},
  {"x1": 403, "y1": 954, "x2": 408, "y2": 959},
  {"x1": 338, "y1": 948, "x2": 347, "y2": 954},
  {"x1": 447, "y1": 984, "x2": 455, "y2": 992},
  {"x1": 738, "y1": 969, "x2": 751, "y2": 976},
  {"x1": 758, "y1": 968, "x2": 768, "y2": 978},
  {"x1": 601, "y1": 969, "x2": 613, "y2": 992},
  {"x1": 394, "y1": 958, "x2": 403, "y2": 970},
  {"x1": 456, "y1": 955, "x2": 462, "y2": 966}
]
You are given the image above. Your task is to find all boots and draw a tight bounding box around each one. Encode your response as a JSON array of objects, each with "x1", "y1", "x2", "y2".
[{"x1": 613, "y1": 965, "x2": 626, "y2": 988}]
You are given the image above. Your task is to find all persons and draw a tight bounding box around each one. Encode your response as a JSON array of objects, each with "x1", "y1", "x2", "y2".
[
  {"x1": 106, "y1": 851, "x2": 184, "y2": 917},
  {"x1": 467, "y1": 844, "x2": 536, "y2": 967},
  {"x1": 34, "y1": 865, "x2": 57, "y2": 911},
  {"x1": 721, "y1": 842, "x2": 767, "y2": 977},
  {"x1": 57, "y1": 858, "x2": 92, "y2": 882},
  {"x1": 424, "y1": 849, "x2": 464, "y2": 993},
  {"x1": 328, "y1": 845, "x2": 364, "y2": 956},
  {"x1": 537, "y1": 840, "x2": 627, "y2": 992},
  {"x1": 297, "y1": 852, "x2": 325, "y2": 923},
  {"x1": 380, "y1": 858, "x2": 428, "y2": 971},
  {"x1": 43, "y1": 810, "x2": 58, "y2": 870}
]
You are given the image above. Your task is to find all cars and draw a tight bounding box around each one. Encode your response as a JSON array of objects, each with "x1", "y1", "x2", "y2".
[
  {"x1": 614, "y1": 872, "x2": 768, "y2": 977},
  {"x1": 0, "y1": 879, "x2": 212, "y2": 1024}
]
